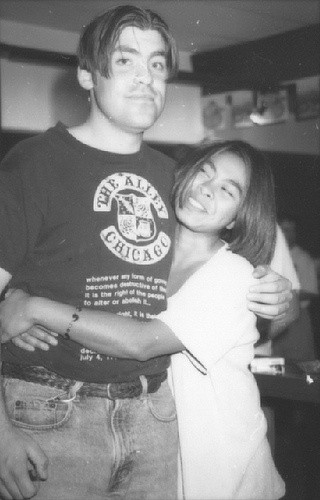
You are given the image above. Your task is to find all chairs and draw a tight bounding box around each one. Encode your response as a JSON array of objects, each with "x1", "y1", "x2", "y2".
[{"x1": 271, "y1": 291, "x2": 320, "y2": 359}]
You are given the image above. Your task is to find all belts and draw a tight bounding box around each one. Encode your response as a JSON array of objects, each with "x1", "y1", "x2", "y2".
[{"x1": 1, "y1": 361, "x2": 168, "y2": 399}]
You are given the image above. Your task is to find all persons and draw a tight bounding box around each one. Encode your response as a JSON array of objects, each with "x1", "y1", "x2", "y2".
[
  {"x1": 2, "y1": 5, "x2": 301, "y2": 500},
  {"x1": 273, "y1": 217, "x2": 319, "y2": 371},
  {"x1": 0, "y1": 138, "x2": 285, "y2": 500}
]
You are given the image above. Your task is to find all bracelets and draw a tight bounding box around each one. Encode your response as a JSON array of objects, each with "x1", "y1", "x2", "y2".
[
  {"x1": 0, "y1": 276, "x2": 17, "y2": 301},
  {"x1": 64, "y1": 304, "x2": 83, "y2": 338}
]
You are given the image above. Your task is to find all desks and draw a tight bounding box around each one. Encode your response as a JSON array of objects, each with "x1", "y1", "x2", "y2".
[{"x1": 247, "y1": 356, "x2": 320, "y2": 403}]
